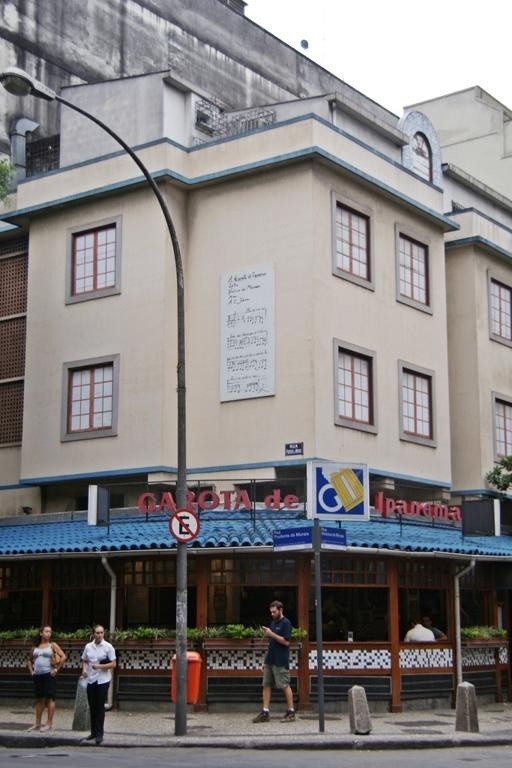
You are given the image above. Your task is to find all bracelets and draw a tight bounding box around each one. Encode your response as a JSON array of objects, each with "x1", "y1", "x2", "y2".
[{"x1": 53, "y1": 667, "x2": 58, "y2": 672}]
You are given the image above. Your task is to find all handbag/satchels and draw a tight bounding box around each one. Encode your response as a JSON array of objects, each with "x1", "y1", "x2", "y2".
[
  {"x1": 99, "y1": 658, "x2": 111, "y2": 664},
  {"x1": 51, "y1": 652, "x2": 60, "y2": 668}
]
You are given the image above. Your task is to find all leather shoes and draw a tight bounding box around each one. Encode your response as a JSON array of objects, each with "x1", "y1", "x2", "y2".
[{"x1": 87, "y1": 735, "x2": 103, "y2": 744}]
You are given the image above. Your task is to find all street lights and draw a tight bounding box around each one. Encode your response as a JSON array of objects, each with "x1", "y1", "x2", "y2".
[{"x1": 0, "y1": 66, "x2": 190, "y2": 740}]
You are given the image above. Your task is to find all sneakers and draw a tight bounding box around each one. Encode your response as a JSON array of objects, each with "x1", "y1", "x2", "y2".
[
  {"x1": 27, "y1": 725, "x2": 52, "y2": 732},
  {"x1": 280, "y1": 711, "x2": 295, "y2": 723},
  {"x1": 253, "y1": 711, "x2": 269, "y2": 723}
]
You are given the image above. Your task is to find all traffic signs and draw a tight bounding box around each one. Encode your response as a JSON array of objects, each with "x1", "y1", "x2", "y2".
[
  {"x1": 272, "y1": 527, "x2": 315, "y2": 554},
  {"x1": 321, "y1": 527, "x2": 348, "y2": 552}
]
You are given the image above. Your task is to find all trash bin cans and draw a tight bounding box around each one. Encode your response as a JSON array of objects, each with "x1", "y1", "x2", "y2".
[{"x1": 170, "y1": 651, "x2": 203, "y2": 704}]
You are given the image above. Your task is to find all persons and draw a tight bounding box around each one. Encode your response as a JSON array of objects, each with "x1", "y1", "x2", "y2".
[
  {"x1": 401, "y1": 613, "x2": 435, "y2": 641},
  {"x1": 24, "y1": 623, "x2": 67, "y2": 732},
  {"x1": 250, "y1": 599, "x2": 297, "y2": 722},
  {"x1": 79, "y1": 623, "x2": 118, "y2": 744},
  {"x1": 420, "y1": 613, "x2": 449, "y2": 641}
]
